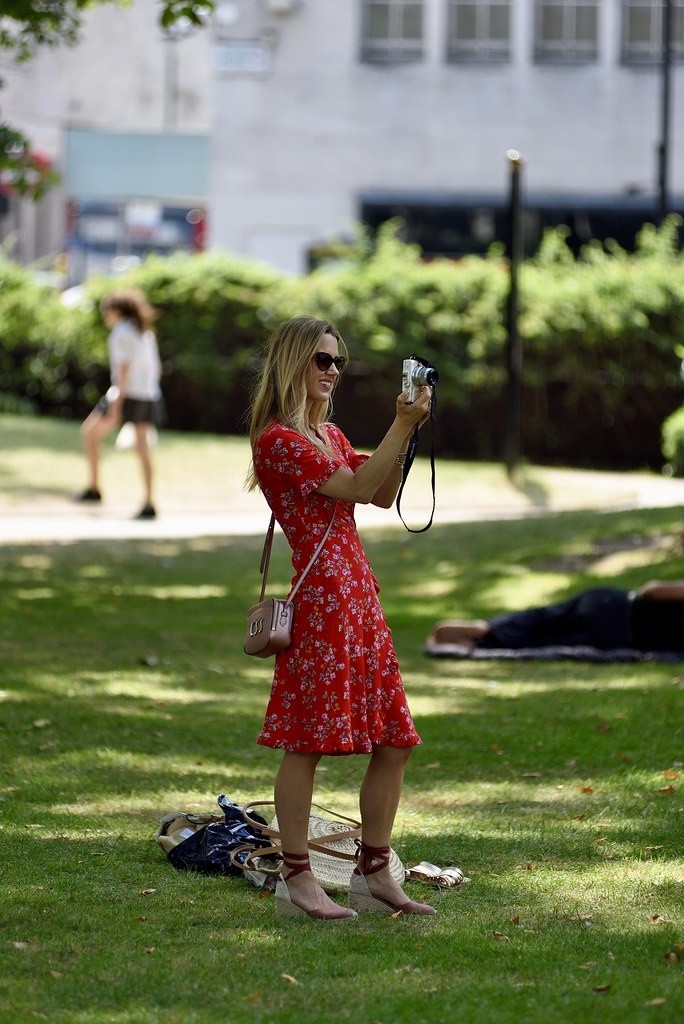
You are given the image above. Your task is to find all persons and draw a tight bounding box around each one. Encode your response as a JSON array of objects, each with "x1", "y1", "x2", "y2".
[
  {"x1": 72, "y1": 286, "x2": 162, "y2": 519},
  {"x1": 242, "y1": 316, "x2": 434, "y2": 923}
]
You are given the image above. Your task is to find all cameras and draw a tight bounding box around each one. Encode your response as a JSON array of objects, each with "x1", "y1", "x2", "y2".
[{"x1": 403, "y1": 360, "x2": 439, "y2": 406}]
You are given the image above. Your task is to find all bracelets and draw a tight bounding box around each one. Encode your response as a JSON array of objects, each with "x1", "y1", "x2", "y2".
[{"x1": 392, "y1": 453, "x2": 406, "y2": 468}]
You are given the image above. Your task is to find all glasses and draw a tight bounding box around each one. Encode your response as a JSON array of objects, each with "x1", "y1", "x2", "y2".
[{"x1": 313, "y1": 352, "x2": 346, "y2": 371}]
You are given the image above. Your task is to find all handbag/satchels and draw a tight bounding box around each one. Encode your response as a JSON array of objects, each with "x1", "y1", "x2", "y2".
[
  {"x1": 230, "y1": 799, "x2": 405, "y2": 893},
  {"x1": 158, "y1": 796, "x2": 269, "y2": 877},
  {"x1": 116, "y1": 422, "x2": 158, "y2": 449},
  {"x1": 244, "y1": 597, "x2": 294, "y2": 658}
]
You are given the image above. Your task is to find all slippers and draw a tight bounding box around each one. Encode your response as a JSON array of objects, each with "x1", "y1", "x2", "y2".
[
  {"x1": 422, "y1": 867, "x2": 471, "y2": 889},
  {"x1": 405, "y1": 861, "x2": 442, "y2": 880}
]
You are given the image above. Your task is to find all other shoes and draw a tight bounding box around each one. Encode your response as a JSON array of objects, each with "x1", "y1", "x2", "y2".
[
  {"x1": 139, "y1": 505, "x2": 156, "y2": 516},
  {"x1": 81, "y1": 489, "x2": 101, "y2": 500}
]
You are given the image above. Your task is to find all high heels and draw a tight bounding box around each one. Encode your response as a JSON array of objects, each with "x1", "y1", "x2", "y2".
[
  {"x1": 347, "y1": 838, "x2": 437, "y2": 915},
  {"x1": 275, "y1": 852, "x2": 358, "y2": 922}
]
